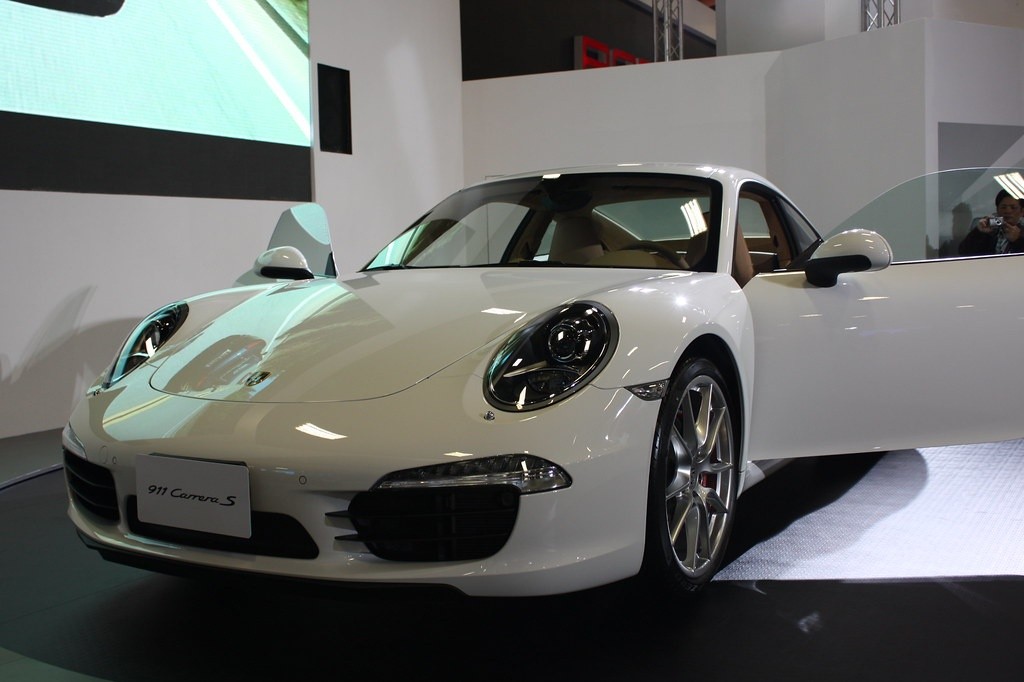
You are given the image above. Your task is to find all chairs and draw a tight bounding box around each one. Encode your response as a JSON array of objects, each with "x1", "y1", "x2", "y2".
[{"x1": 686, "y1": 212, "x2": 755, "y2": 288}]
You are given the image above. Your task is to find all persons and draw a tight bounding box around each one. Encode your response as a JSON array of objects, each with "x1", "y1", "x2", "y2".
[{"x1": 959, "y1": 187, "x2": 1024, "y2": 256}]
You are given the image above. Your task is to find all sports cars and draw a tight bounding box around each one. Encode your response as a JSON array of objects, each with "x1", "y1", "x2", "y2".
[{"x1": 59, "y1": 164, "x2": 1024, "y2": 611}]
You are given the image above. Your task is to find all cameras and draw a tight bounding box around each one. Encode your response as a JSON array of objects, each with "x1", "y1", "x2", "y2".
[{"x1": 989, "y1": 216, "x2": 1005, "y2": 226}]
